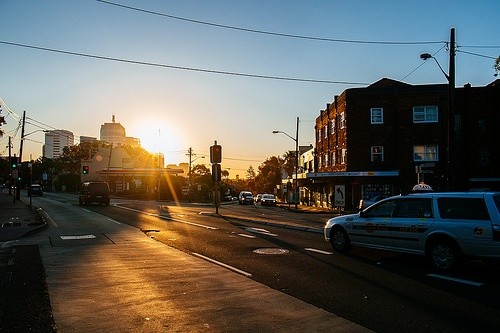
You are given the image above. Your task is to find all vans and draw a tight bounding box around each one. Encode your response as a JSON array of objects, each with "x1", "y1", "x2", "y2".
[
  {"x1": 239, "y1": 191, "x2": 254, "y2": 205},
  {"x1": 79, "y1": 182, "x2": 111, "y2": 206}
]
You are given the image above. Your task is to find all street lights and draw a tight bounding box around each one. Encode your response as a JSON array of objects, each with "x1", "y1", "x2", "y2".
[
  {"x1": 419, "y1": 53, "x2": 449, "y2": 81},
  {"x1": 23, "y1": 130, "x2": 49, "y2": 137},
  {"x1": 191, "y1": 156, "x2": 205, "y2": 163},
  {"x1": 272, "y1": 131, "x2": 296, "y2": 141}
]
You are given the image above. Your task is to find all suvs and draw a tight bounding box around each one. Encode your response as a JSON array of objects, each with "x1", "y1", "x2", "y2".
[
  {"x1": 261, "y1": 194, "x2": 276, "y2": 206},
  {"x1": 28, "y1": 184, "x2": 43, "y2": 197}
]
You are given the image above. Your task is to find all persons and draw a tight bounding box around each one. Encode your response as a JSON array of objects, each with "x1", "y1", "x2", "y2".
[
  {"x1": 301, "y1": 196, "x2": 305, "y2": 204},
  {"x1": 357, "y1": 197, "x2": 365, "y2": 210},
  {"x1": 241, "y1": 193, "x2": 246, "y2": 200}
]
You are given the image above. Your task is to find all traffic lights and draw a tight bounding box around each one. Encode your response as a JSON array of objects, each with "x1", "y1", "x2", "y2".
[
  {"x1": 12, "y1": 164, "x2": 17, "y2": 168},
  {"x1": 82, "y1": 166, "x2": 89, "y2": 174},
  {"x1": 17, "y1": 177, "x2": 22, "y2": 182},
  {"x1": 210, "y1": 145, "x2": 222, "y2": 163}
]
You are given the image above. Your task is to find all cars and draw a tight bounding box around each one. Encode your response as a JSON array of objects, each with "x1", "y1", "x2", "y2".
[{"x1": 324, "y1": 184, "x2": 500, "y2": 271}]
